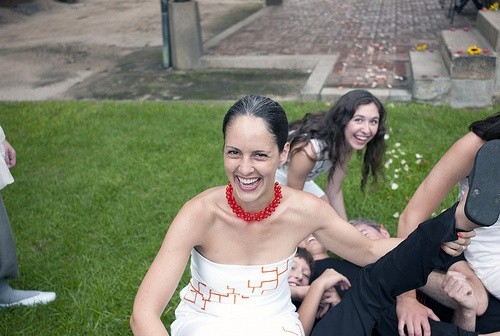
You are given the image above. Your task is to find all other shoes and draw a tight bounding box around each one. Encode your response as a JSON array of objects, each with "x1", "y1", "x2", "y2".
[{"x1": 0, "y1": 289, "x2": 56, "y2": 308}]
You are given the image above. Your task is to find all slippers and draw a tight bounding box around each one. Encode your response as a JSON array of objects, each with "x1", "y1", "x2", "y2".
[{"x1": 464, "y1": 139, "x2": 500, "y2": 228}]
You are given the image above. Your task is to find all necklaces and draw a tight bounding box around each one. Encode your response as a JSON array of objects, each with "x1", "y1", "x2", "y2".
[{"x1": 226, "y1": 181, "x2": 283, "y2": 222}]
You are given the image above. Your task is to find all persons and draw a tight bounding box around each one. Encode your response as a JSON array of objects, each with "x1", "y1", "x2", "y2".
[
  {"x1": 275, "y1": 88, "x2": 500, "y2": 336},
  {"x1": 0, "y1": 124, "x2": 57, "y2": 308},
  {"x1": 130, "y1": 94, "x2": 475, "y2": 336}
]
